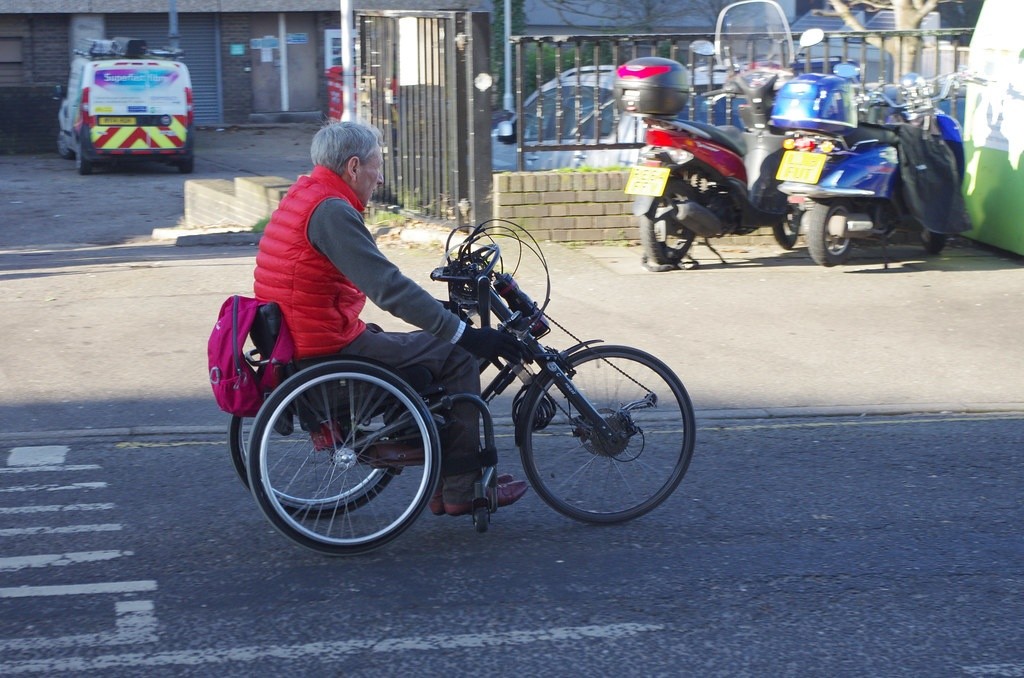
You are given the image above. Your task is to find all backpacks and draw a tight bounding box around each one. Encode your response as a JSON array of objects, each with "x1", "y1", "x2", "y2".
[{"x1": 207, "y1": 295, "x2": 295, "y2": 417}]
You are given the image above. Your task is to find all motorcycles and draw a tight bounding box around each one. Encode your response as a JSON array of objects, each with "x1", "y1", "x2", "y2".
[
  {"x1": 608, "y1": 0, "x2": 826, "y2": 267},
  {"x1": 765, "y1": 58, "x2": 989, "y2": 270}
]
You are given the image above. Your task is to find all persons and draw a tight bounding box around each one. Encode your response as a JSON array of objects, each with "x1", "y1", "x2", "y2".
[{"x1": 253, "y1": 120, "x2": 532, "y2": 518}]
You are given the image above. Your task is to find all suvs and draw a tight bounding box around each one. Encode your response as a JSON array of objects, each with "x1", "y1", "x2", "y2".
[
  {"x1": 676, "y1": 64, "x2": 753, "y2": 134},
  {"x1": 490, "y1": 65, "x2": 654, "y2": 176}
]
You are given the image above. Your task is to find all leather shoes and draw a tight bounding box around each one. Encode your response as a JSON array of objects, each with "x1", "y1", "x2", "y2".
[{"x1": 430, "y1": 474, "x2": 529, "y2": 516}]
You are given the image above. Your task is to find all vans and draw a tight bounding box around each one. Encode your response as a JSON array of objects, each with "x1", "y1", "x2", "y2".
[{"x1": 53, "y1": 36, "x2": 196, "y2": 176}]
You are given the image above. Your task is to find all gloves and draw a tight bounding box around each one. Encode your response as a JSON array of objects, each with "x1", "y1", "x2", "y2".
[
  {"x1": 456, "y1": 322, "x2": 525, "y2": 370},
  {"x1": 435, "y1": 299, "x2": 474, "y2": 326}
]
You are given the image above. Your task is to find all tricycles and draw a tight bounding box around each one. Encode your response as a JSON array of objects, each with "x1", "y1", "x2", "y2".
[{"x1": 230, "y1": 215, "x2": 698, "y2": 557}]
